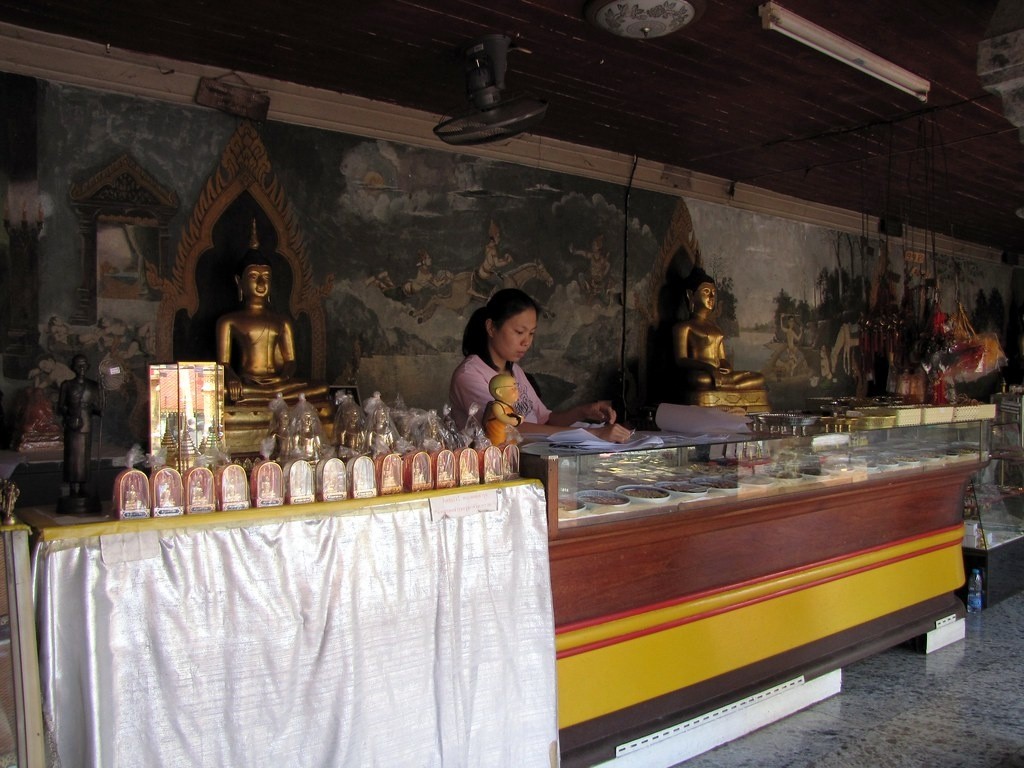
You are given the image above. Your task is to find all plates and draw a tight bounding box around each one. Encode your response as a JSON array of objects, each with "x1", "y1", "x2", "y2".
[{"x1": 557, "y1": 438, "x2": 978, "y2": 517}]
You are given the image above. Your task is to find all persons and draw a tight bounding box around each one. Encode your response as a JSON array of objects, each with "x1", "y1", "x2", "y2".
[
  {"x1": 0, "y1": 215, "x2": 1006, "y2": 521},
  {"x1": 450, "y1": 288, "x2": 631, "y2": 445}
]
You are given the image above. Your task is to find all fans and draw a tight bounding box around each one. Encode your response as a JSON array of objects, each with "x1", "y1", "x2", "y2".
[{"x1": 433, "y1": 43, "x2": 549, "y2": 145}]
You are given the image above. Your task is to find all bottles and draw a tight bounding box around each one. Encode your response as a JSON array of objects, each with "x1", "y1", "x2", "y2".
[{"x1": 967, "y1": 568, "x2": 982, "y2": 612}]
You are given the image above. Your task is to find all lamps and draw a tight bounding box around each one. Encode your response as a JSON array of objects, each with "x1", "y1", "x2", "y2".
[
  {"x1": 585, "y1": 0, "x2": 697, "y2": 40},
  {"x1": 758, "y1": 1, "x2": 930, "y2": 104}
]
